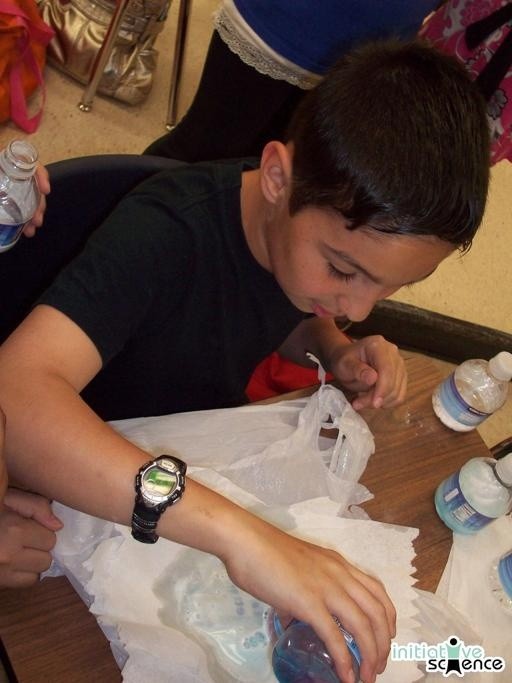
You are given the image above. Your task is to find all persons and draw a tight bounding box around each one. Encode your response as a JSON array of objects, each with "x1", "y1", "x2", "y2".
[
  {"x1": 140, "y1": 0, "x2": 451, "y2": 165},
  {"x1": 1, "y1": 139, "x2": 53, "y2": 241},
  {"x1": 1, "y1": 36, "x2": 490, "y2": 682},
  {"x1": 0, "y1": 407, "x2": 65, "y2": 594}
]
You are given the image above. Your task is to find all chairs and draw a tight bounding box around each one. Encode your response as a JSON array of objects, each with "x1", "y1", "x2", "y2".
[{"x1": 2, "y1": 153, "x2": 191, "y2": 334}]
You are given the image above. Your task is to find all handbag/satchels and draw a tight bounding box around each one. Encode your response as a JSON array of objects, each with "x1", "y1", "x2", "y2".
[
  {"x1": 37, "y1": 0, "x2": 174, "y2": 107},
  {"x1": 0, "y1": 0, "x2": 58, "y2": 133}
]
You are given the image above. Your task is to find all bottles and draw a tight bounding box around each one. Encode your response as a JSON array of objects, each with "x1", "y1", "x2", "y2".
[
  {"x1": 433, "y1": 450, "x2": 512, "y2": 536},
  {"x1": 430, "y1": 347, "x2": 512, "y2": 434},
  {"x1": 487, "y1": 547, "x2": 512, "y2": 614},
  {"x1": 1, "y1": 140, "x2": 43, "y2": 257},
  {"x1": 270, "y1": 614, "x2": 360, "y2": 682}
]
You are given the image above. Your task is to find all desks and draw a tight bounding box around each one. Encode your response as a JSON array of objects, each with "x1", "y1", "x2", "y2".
[{"x1": 2, "y1": 350, "x2": 496, "y2": 680}]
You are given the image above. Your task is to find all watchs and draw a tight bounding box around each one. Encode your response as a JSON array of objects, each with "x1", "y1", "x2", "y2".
[{"x1": 130, "y1": 452, "x2": 188, "y2": 544}]
